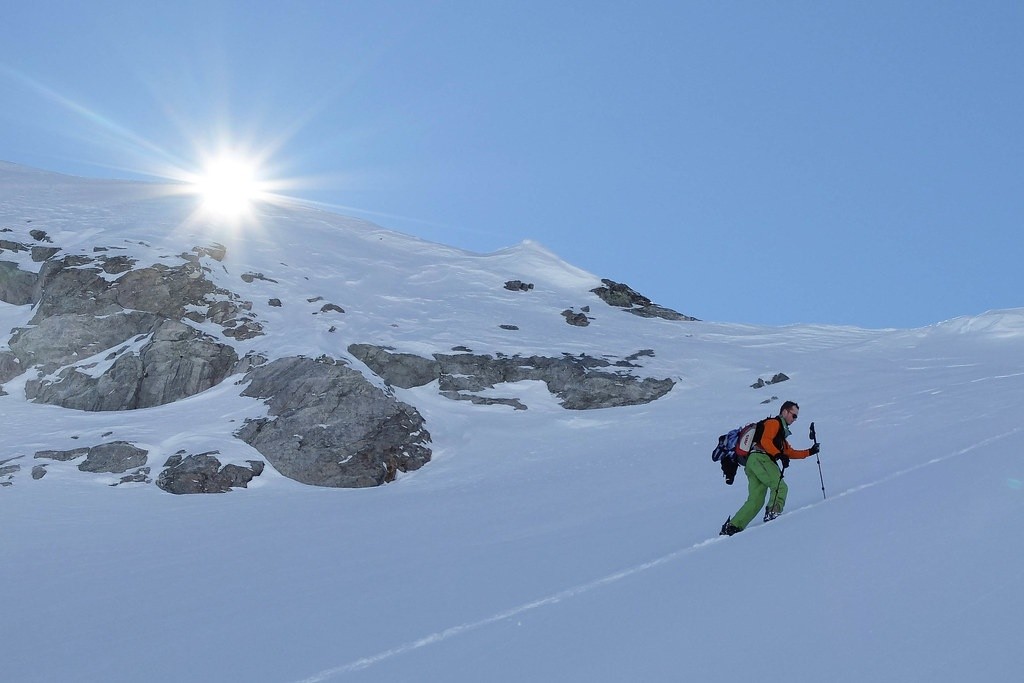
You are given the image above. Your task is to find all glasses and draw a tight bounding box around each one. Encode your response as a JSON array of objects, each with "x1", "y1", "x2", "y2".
[{"x1": 787, "y1": 409, "x2": 797, "y2": 418}]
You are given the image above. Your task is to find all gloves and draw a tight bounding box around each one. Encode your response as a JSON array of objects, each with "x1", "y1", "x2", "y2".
[
  {"x1": 810, "y1": 443, "x2": 820, "y2": 455},
  {"x1": 780, "y1": 454, "x2": 789, "y2": 468}
]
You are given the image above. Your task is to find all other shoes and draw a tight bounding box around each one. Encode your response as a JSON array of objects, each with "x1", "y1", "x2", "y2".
[
  {"x1": 719, "y1": 516, "x2": 742, "y2": 537},
  {"x1": 764, "y1": 512, "x2": 782, "y2": 521}
]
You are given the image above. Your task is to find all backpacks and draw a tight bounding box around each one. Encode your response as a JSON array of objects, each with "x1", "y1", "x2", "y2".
[{"x1": 736, "y1": 417, "x2": 781, "y2": 466}]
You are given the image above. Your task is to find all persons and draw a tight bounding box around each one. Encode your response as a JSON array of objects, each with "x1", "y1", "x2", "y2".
[{"x1": 719, "y1": 400, "x2": 820, "y2": 536}]
